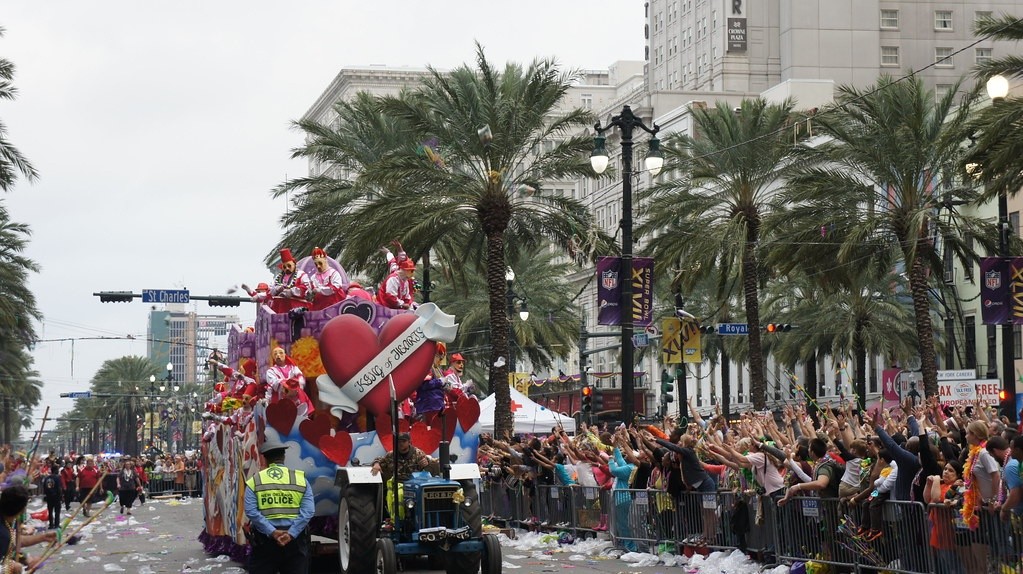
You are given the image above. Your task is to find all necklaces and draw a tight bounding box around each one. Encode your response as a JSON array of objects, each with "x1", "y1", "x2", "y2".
[{"x1": 4, "y1": 520, "x2": 15, "y2": 574}]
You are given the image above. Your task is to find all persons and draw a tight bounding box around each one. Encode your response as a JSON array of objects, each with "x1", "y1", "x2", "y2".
[
  {"x1": 370, "y1": 433, "x2": 434, "y2": 526},
  {"x1": 75, "y1": 458, "x2": 104, "y2": 517},
  {"x1": 198, "y1": 357, "x2": 261, "y2": 443},
  {"x1": 44, "y1": 466, "x2": 63, "y2": 528},
  {"x1": 0, "y1": 442, "x2": 59, "y2": 574},
  {"x1": 244, "y1": 247, "x2": 344, "y2": 315},
  {"x1": 634, "y1": 393, "x2": 1023, "y2": 574},
  {"x1": 397, "y1": 341, "x2": 474, "y2": 422},
  {"x1": 242, "y1": 443, "x2": 315, "y2": 574},
  {"x1": 55, "y1": 442, "x2": 203, "y2": 500},
  {"x1": 478, "y1": 415, "x2": 649, "y2": 554},
  {"x1": 265, "y1": 346, "x2": 316, "y2": 420},
  {"x1": 115, "y1": 460, "x2": 141, "y2": 515},
  {"x1": 61, "y1": 461, "x2": 75, "y2": 511},
  {"x1": 347, "y1": 241, "x2": 416, "y2": 308}
]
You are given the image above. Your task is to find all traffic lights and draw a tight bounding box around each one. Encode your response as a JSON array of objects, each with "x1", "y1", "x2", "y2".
[
  {"x1": 591, "y1": 385, "x2": 604, "y2": 411},
  {"x1": 581, "y1": 386, "x2": 592, "y2": 414},
  {"x1": 999, "y1": 388, "x2": 1009, "y2": 418},
  {"x1": 768, "y1": 323, "x2": 792, "y2": 332},
  {"x1": 698, "y1": 324, "x2": 713, "y2": 335},
  {"x1": 660, "y1": 369, "x2": 676, "y2": 404}
]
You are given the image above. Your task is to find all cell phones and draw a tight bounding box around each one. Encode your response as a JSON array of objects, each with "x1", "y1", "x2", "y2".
[{"x1": 867, "y1": 435, "x2": 872, "y2": 446}]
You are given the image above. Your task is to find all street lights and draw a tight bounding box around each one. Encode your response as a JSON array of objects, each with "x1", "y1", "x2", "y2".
[
  {"x1": 142, "y1": 376, "x2": 161, "y2": 448},
  {"x1": 203, "y1": 348, "x2": 227, "y2": 390},
  {"x1": 159, "y1": 361, "x2": 181, "y2": 452},
  {"x1": 587, "y1": 101, "x2": 665, "y2": 449},
  {"x1": 906, "y1": 369, "x2": 921, "y2": 406},
  {"x1": 502, "y1": 264, "x2": 531, "y2": 375},
  {"x1": 985, "y1": 71, "x2": 1019, "y2": 435}
]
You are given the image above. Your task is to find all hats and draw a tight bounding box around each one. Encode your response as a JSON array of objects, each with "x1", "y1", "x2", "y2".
[
  {"x1": 398, "y1": 432, "x2": 410, "y2": 441},
  {"x1": 400, "y1": 258, "x2": 416, "y2": 270},
  {"x1": 312, "y1": 246, "x2": 327, "y2": 261},
  {"x1": 435, "y1": 343, "x2": 446, "y2": 359},
  {"x1": 255, "y1": 283, "x2": 268, "y2": 291},
  {"x1": 259, "y1": 440, "x2": 289, "y2": 457},
  {"x1": 450, "y1": 353, "x2": 464, "y2": 362},
  {"x1": 174, "y1": 454, "x2": 182, "y2": 459},
  {"x1": 279, "y1": 248, "x2": 293, "y2": 263}
]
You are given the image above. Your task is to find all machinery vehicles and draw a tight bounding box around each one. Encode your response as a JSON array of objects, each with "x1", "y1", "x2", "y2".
[{"x1": 193, "y1": 370, "x2": 504, "y2": 574}]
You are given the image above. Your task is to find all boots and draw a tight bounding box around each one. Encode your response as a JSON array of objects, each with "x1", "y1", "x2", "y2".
[{"x1": 593, "y1": 514, "x2": 609, "y2": 531}]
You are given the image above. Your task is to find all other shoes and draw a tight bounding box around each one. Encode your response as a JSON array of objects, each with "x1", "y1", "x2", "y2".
[
  {"x1": 55, "y1": 525, "x2": 62, "y2": 528},
  {"x1": 120, "y1": 508, "x2": 124, "y2": 514},
  {"x1": 86, "y1": 512, "x2": 90, "y2": 517},
  {"x1": 83, "y1": 510, "x2": 86, "y2": 516},
  {"x1": 48, "y1": 526, "x2": 54, "y2": 529},
  {"x1": 127, "y1": 512, "x2": 131, "y2": 515},
  {"x1": 495, "y1": 512, "x2": 571, "y2": 526}
]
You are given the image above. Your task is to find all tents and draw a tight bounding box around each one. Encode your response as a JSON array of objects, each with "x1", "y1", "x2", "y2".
[{"x1": 477, "y1": 386, "x2": 576, "y2": 436}]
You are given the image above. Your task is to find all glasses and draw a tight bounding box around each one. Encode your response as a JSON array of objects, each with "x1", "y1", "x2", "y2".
[{"x1": 457, "y1": 361, "x2": 463, "y2": 364}]
